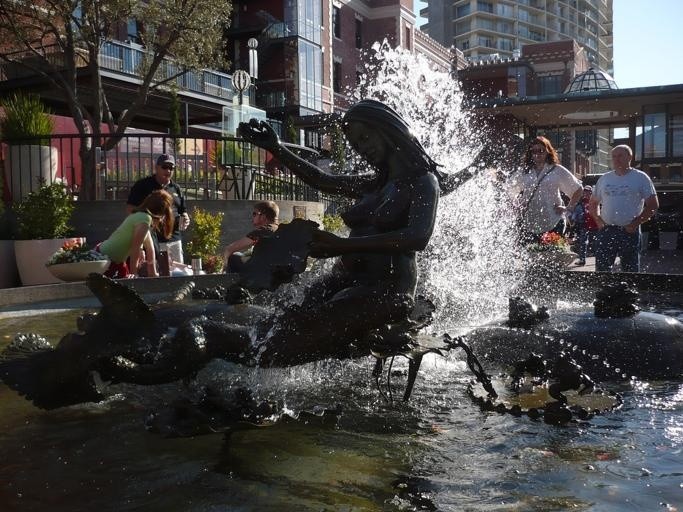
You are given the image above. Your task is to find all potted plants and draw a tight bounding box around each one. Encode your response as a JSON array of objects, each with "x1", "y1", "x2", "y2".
[
  {"x1": 0, "y1": 94, "x2": 58, "y2": 205},
  {"x1": 13, "y1": 176, "x2": 87, "y2": 286},
  {"x1": 209, "y1": 134, "x2": 255, "y2": 200}
]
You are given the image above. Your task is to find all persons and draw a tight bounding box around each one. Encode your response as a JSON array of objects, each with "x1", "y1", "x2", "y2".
[
  {"x1": 588, "y1": 144, "x2": 659, "y2": 271},
  {"x1": 126, "y1": 154, "x2": 191, "y2": 271},
  {"x1": 571, "y1": 197, "x2": 584, "y2": 237},
  {"x1": 94, "y1": 190, "x2": 172, "y2": 279},
  {"x1": 221, "y1": 200, "x2": 279, "y2": 275},
  {"x1": 574, "y1": 185, "x2": 600, "y2": 266},
  {"x1": 238, "y1": 99, "x2": 444, "y2": 368},
  {"x1": 503, "y1": 137, "x2": 583, "y2": 248}
]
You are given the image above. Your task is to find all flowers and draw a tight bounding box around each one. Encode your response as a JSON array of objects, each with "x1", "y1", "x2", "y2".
[{"x1": 45, "y1": 237, "x2": 108, "y2": 268}]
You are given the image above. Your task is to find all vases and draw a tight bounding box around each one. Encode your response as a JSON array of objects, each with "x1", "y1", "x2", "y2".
[{"x1": 47, "y1": 260, "x2": 112, "y2": 286}]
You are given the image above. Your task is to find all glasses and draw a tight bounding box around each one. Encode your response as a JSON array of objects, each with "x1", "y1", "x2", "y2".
[
  {"x1": 252, "y1": 211, "x2": 262, "y2": 217},
  {"x1": 532, "y1": 148, "x2": 546, "y2": 154},
  {"x1": 160, "y1": 163, "x2": 175, "y2": 170}
]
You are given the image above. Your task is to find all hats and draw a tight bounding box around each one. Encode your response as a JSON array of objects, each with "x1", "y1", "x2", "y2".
[
  {"x1": 583, "y1": 184, "x2": 593, "y2": 192},
  {"x1": 157, "y1": 153, "x2": 175, "y2": 165}
]
